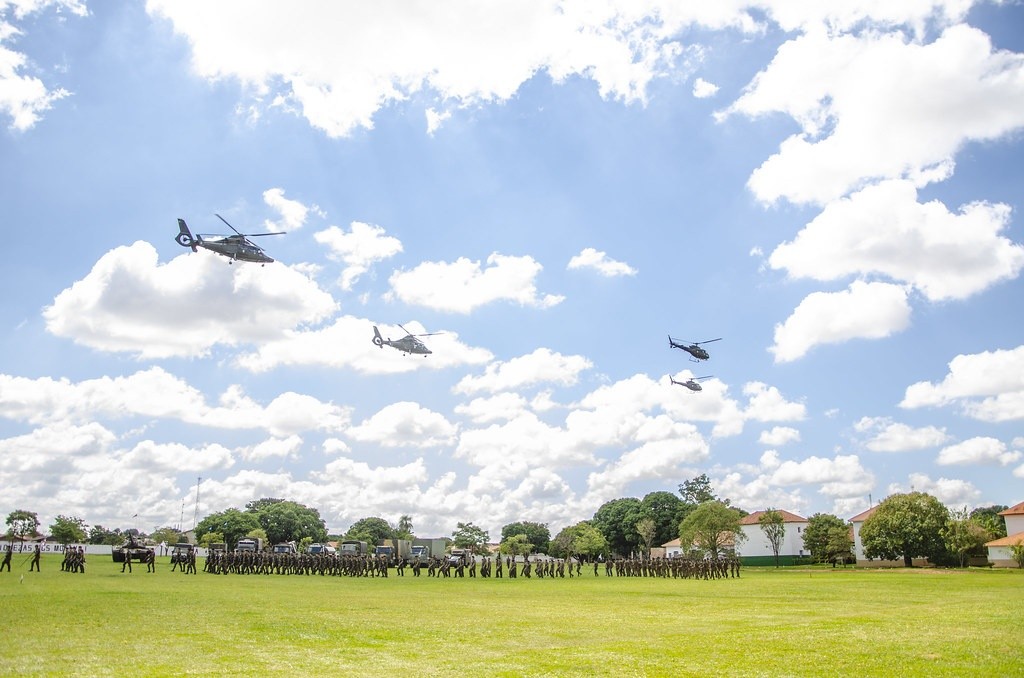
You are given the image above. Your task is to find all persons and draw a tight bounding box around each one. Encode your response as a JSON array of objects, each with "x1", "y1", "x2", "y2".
[
  {"x1": 0, "y1": 545, "x2": 12, "y2": 572},
  {"x1": 29, "y1": 545, "x2": 40, "y2": 572},
  {"x1": 509, "y1": 556, "x2": 740, "y2": 580},
  {"x1": 60, "y1": 546, "x2": 86, "y2": 573},
  {"x1": 171, "y1": 548, "x2": 182, "y2": 572},
  {"x1": 412, "y1": 555, "x2": 503, "y2": 578},
  {"x1": 147, "y1": 548, "x2": 155, "y2": 573},
  {"x1": 397, "y1": 555, "x2": 405, "y2": 576},
  {"x1": 182, "y1": 548, "x2": 196, "y2": 574},
  {"x1": 120, "y1": 549, "x2": 132, "y2": 573},
  {"x1": 202, "y1": 547, "x2": 388, "y2": 578},
  {"x1": 832, "y1": 556, "x2": 837, "y2": 568},
  {"x1": 507, "y1": 556, "x2": 510, "y2": 569}
]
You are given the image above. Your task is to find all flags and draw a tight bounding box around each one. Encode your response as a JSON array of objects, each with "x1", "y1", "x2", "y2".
[{"x1": 39, "y1": 539, "x2": 46, "y2": 551}]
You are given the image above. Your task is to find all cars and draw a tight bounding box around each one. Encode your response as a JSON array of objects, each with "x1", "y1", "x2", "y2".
[{"x1": 549, "y1": 558, "x2": 555, "y2": 563}]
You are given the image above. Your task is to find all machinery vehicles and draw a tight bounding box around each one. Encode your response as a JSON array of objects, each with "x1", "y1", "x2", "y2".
[{"x1": 111, "y1": 532, "x2": 155, "y2": 563}]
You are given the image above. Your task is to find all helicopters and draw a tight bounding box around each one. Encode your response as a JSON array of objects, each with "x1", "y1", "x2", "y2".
[
  {"x1": 372, "y1": 323, "x2": 444, "y2": 359},
  {"x1": 669, "y1": 375, "x2": 713, "y2": 393},
  {"x1": 668, "y1": 334, "x2": 722, "y2": 363},
  {"x1": 175, "y1": 214, "x2": 287, "y2": 267}
]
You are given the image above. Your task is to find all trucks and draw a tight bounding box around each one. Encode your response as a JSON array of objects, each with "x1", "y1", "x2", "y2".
[
  {"x1": 171, "y1": 543, "x2": 194, "y2": 563},
  {"x1": 409, "y1": 538, "x2": 445, "y2": 568},
  {"x1": 375, "y1": 540, "x2": 410, "y2": 568},
  {"x1": 237, "y1": 536, "x2": 263, "y2": 555},
  {"x1": 308, "y1": 543, "x2": 336, "y2": 557},
  {"x1": 208, "y1": 543, "x2": 233, "y2": 556},
  {"x1": 339, "y1": 540, "x2": 368, "y2": 559},
  {"x1": 271, "y1": 541, "x2": 297, "y2": 556},
  {"x1": 447, "y1": 549, "x2": 472, "y2": 568}
]
